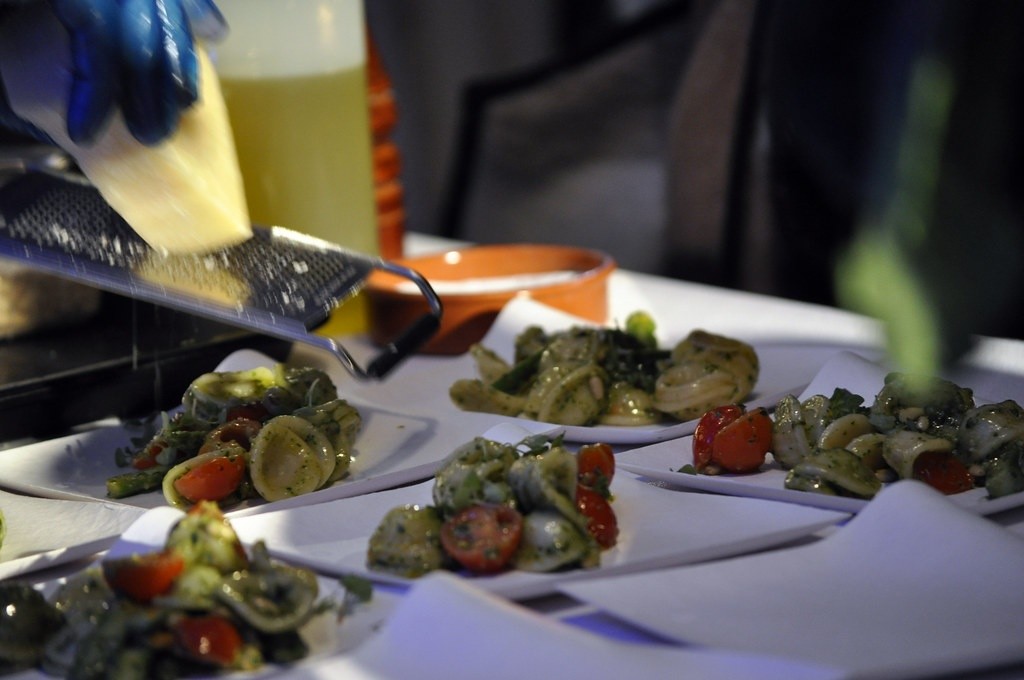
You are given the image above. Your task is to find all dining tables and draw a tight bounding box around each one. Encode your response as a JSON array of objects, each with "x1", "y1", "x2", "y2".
[{"x1": 1, "y1": 229, "x2": 1024, "y2": 679}]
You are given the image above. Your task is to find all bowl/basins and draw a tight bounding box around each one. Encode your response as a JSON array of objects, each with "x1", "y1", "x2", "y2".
[{"x1": 358, "y1": 244, "x2": 616, "y2": 355}]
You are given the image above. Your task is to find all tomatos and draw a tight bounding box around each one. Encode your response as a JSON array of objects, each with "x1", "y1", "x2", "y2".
[
  {"x1": 693, "y1": 403, "x2": 778, "y2": 472},
  {"x1": 173, "y1": 456, "x2": 247, "y2": 508},
  {"x1": 99, "y1": 550, "x2": 244, "y2": 666},
  {"x1": 442, "y1": 442, "x2": 620, "y2": 569}
]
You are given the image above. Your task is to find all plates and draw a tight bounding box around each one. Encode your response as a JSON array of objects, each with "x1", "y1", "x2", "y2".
[{"x1": 0, "y1": 293, "x2": 1023, "y2": 680}]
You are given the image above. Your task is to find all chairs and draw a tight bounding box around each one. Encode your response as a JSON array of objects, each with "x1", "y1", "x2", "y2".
[{"x1": 431, "y1": 0, "x2": 1024, "y2": 345}]
